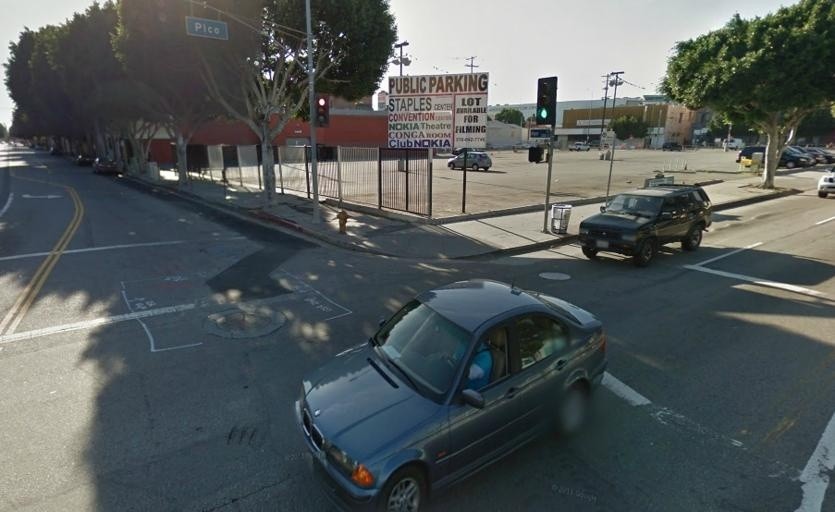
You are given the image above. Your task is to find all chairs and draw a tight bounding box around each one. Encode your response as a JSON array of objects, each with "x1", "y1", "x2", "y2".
[{"x1": 482, "y1": 333, "x2": 507, "y2": 380}]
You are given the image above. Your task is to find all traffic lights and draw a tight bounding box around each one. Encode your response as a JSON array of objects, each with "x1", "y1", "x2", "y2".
[
  {"x1": 314, "y1": 93, "x2": 330, "y2": 128},
  {"x1": 536, "y1": 76, "x2": 558, "y2": 127}
]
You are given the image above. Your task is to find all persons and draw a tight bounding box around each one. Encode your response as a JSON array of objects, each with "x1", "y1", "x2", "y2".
[{"x1": 445, "y1": 331, "x2": 495, "y2": 395}]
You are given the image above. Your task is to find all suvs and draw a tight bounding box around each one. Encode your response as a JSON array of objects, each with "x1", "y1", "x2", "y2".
[
  {"x1": 579, "y1": 183, "x2": 714, "y2": 265},
  {"x1": 662, "y1": 142, "x2": 682, "y2": 152}
]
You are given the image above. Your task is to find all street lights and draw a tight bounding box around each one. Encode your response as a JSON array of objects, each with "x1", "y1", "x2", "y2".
[{"x1": 394, "y1": 42, "x2": 410, "y2": 77}]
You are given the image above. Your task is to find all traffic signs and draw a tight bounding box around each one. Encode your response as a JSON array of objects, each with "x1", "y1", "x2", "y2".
[{"x1": 531, "y1": 129, "x2": 551, "y2": 138}]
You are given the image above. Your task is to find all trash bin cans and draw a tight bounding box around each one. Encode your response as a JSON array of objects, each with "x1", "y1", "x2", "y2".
[
  {"x1": 528, "y1": 146, "x2": 544, "y2": 163},
  {"x1": 551, "y1": 204, "x2": 572, "y2": 234}
]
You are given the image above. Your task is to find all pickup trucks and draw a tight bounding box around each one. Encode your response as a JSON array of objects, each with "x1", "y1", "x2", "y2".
[{"x1": 569, "y1": 141, "x2": 590, "y2": 152}]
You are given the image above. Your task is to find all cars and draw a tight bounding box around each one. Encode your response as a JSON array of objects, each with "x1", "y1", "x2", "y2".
[
  {"x1": 286, "y1": 276, "x2": 607, "y2": 510},
  {"x1": 448, "y1": 148, "x2": 494, "y2": 172},
  {"x1": 817, "y1": 164, "x2": 835, "y2": 198},
  {"x1": 723, "y1": 136, "x2": 835, "y2": 168},
  {"x1": 1, "y1": 139, "x2": 117, "y2": 175}
]
